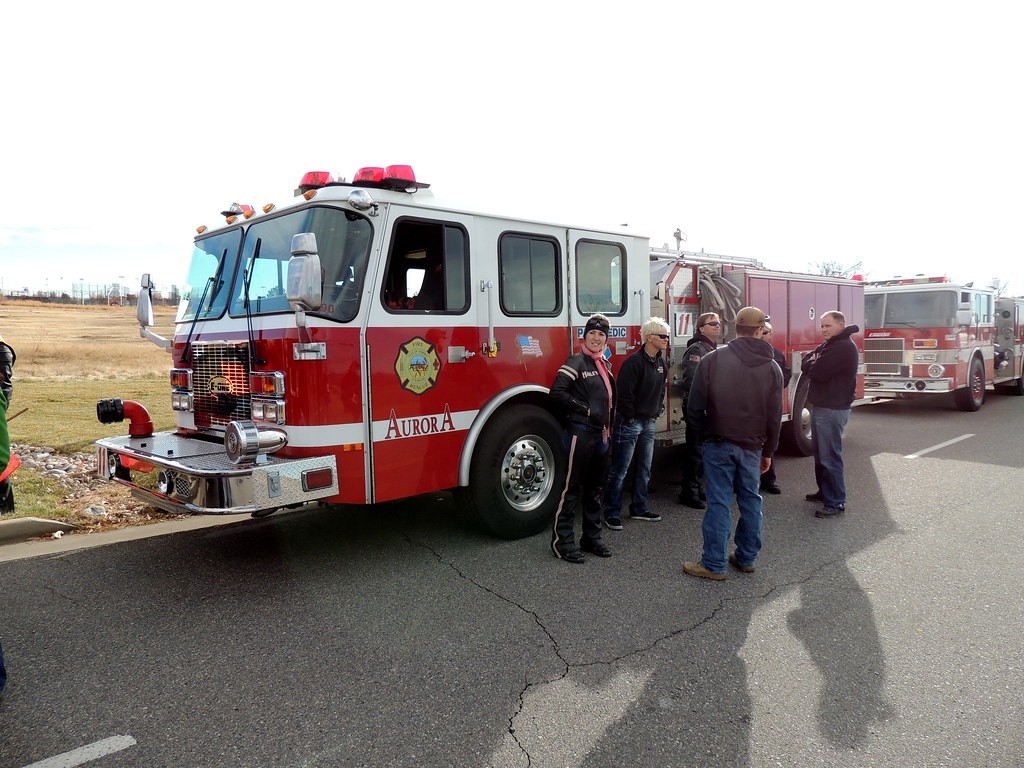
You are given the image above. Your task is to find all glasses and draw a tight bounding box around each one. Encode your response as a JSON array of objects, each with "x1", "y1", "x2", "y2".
[
  {"x1": 652, "y1": 333, "x2": 670, "y2": 340},
  {"x1": 763, "y1": 331, "x2": 771, "y2": 335},
  {"x1": 703, "y1": 321, "x2": 722, "y2": 327},
  {"x1": 588, "y1": 319, "x2": 608, "y2": 329}
]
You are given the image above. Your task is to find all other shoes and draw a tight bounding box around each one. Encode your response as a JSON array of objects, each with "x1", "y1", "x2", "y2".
[
  {"x1": 697, "y1": 488, "x2": 708, "y2": 500},
  {"x1": 0, "y1": 503, "x2": 16, "y2": 515},
  {"x1": 580, "y1": 539, "x2": 612, "y2": 557},
  {"x1": 759, "y1": 480, "x2": 782, "y2": 493},
  {"x1": 678, "y1": 494, "x2": 707, "y2": 509},
  {"x1": 562, "y1": 550, "x2": 587, "y2": 563}
]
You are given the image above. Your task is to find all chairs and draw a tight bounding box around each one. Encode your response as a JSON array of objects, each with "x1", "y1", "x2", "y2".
[{"x1": 417, "y1": 244, "x2": 445, "y2": 310}]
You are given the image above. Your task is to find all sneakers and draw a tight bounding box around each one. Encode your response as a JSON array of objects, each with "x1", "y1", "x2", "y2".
[
  {"x1": 815, "y1": 505, "x2": 845, "y2": 517},
  {"x1": 684, "y1": 561, "x2": 727, "y2": 580},
  {"x1": 632, "y1": 511, "x2": 662, "y2": 521},
  {"x1": 603, "y1": 516, "x2": 624, "y2": 530},
  {"x1": 729, "y1": 551, "x2": 755, "y2": 571},
  {"x1": 806, "y1": 492, "x2": 826, "y2": 502}
]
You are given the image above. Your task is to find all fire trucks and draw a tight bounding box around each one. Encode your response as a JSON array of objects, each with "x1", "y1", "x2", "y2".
[
  {"x1": 861, "y1": 273, "x2": 1024, "y2": 412},
  {"x1": 91, "y1": 165, "x2": 874, "y2": 540}
]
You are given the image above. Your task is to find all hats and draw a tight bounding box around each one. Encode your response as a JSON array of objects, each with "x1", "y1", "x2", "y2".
[{"x1": 736, "y1": 306, "x2": 765, "y2": 326}]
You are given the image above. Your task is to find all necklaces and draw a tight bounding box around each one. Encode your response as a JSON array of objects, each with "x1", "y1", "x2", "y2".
[{"x1": 645, "y1": 349, "x2": 656, "y2": 362}]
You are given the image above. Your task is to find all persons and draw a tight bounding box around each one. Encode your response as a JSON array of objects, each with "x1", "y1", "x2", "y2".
[
  {"x1": 801, "y1": 310, "x2": 859, "y2": 517},
  {"x1": 603, "y1": 318, "x2": 671, "y2": 530},
  {"x1": 549, "y1": 314, "x2": 618, "y2": 563},
  {"x1": 683, "y1": 307, "x2": 783, "y2": 580},
  {"x1": 760, "y1": 321, "x2": 791, "y2": 494},
  {"x1": 0, "y1": 335, "x2": 16, "y2": 514},
  {"x1": 679, "y1": 313, "x2": 721, "y2": 510}
]
